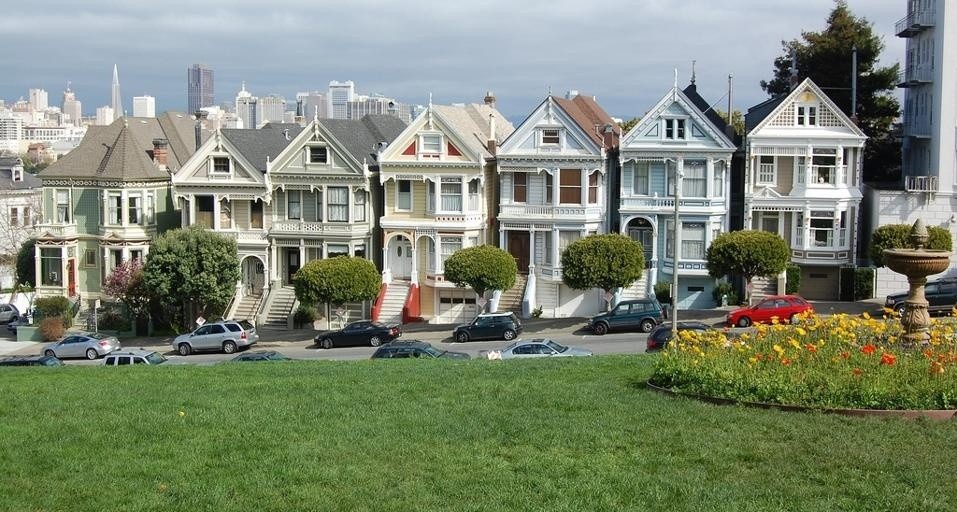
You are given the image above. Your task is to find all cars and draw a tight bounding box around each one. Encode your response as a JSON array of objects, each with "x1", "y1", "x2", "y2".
[
  {"x1": 727, "y1": 295, "x2": 815, "y2": 324},
  {"x1": 173, "y1": 320, "x2": 258, "y2": 353},
  {"x1": 314, "y1": 320, "x2": 402, "y2": 347},
  {"x1": 645, "y1": 322, "x2": 740, "y2": 353},
  {"x1": 0, "y1": 303, "x2": 28, "y2": 334},
  {"x1": 231, "y1": 351, "x2": 295, "y2": 362},
  {"x1": 500, "y1": 338, "x2": 592, "y2": 356},
  {"x1": 40, "y1": 332, "x2": 120, "y2": 360}
]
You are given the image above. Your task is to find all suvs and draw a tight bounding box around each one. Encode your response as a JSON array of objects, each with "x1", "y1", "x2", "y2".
[
  {"x1": 453, "y1": 312, "x2": 522, "y2": 340},
  {"x1": 587, "y1": 298, "x2": 664, "y2": 332},
  {"x1": 371, "y1": 340, "x2": 470, "y2": 359},
  {"x1": 885, "y1": 277, "x2": 956, "y2": 318},
  {"x1": 101, "y1": 348, "x2": 190, "y2": 366}
]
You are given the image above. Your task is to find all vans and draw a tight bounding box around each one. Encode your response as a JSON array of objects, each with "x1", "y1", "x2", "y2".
[{"x1": 0, "y1": 356, "x2": 63, "y2": 366}]
